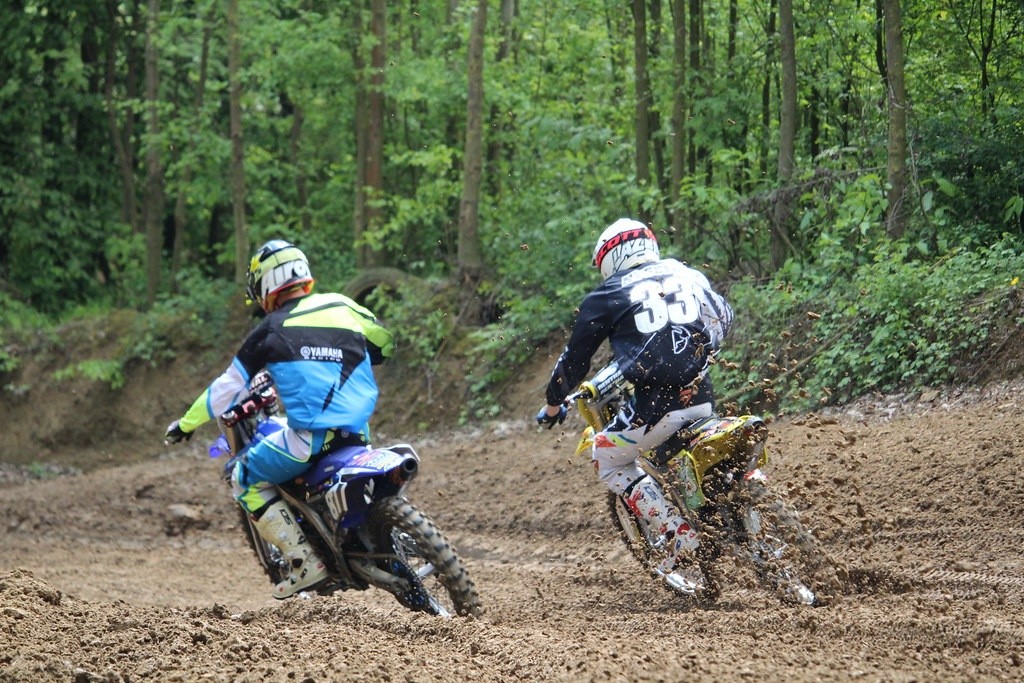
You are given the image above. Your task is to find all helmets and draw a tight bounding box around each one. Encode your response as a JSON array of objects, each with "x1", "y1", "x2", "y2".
[
  {"x1": 591, "y1": 218, "x2": 660, "y2": 279},
  {"x1": 245, "y1": 239, "x2": 314, "y2": 312}
]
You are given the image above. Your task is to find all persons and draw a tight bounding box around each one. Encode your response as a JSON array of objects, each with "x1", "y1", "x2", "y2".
[
  {"x1": 164, "y1": 240, "x2": 395, "y2": 601},
  {"x1": 532, "y1": 217, "x2": 769, "y2": 570}
]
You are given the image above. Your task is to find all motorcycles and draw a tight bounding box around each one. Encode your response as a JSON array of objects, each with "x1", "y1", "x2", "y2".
[
  {"x1": 161, "y1": 372, "x2": 490, "y2": 628},
  {"x1": 533, "y1": 356, "x2": 843, "y2": 612}
]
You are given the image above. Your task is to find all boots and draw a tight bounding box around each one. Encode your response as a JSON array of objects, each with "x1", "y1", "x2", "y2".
[
  {"x1": 250, "y1": 495, "x2": 329, "y2": 600},
  {"x1": 623, "y1": 474, "x2": 699, "y2": 574}
]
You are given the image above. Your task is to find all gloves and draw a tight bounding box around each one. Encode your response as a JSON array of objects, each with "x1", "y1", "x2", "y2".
[
  {"x1": 165, "y1": 420, "x2": 196, "y2": 446},
  {"x1": 537, "y1": 403, "x2": 567, "y2": 430}
]
